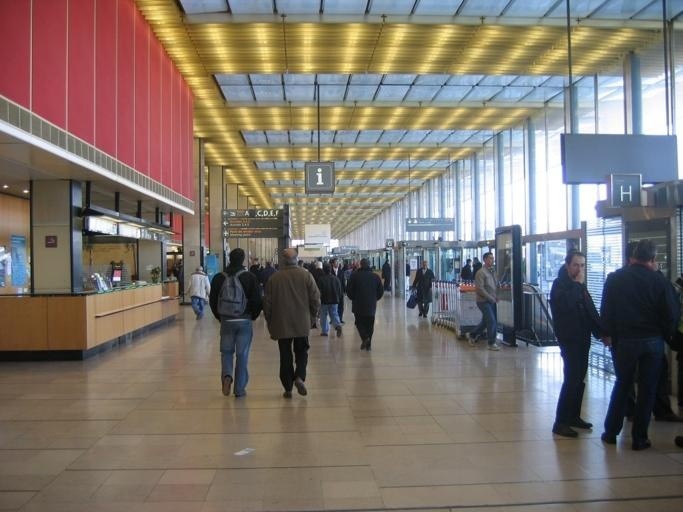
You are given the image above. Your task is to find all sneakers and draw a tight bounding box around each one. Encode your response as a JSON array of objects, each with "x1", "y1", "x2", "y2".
[
  {"x1": 600, "y1": 405, "x2": 683, "y2": 454},
  {"x1": 465, "y1": 332, "x2": 503, "y2": 353},
  {"x1": 233, "y1": 390, "x2": 248, "y2": 398},
  {"x1": 320, "y1": 331, "x2": 329, "y2": 337},
  {"x1": 418, "y1": 312, "x2": 429, "y2": 318},
  {"x1": 335, "y1": 326, "x2": 343, "y2": 338},
  {"x1": 551, "y1": 421, "x2": 578, "y2": 438},
  {"x1": 282, "y1": 389, "x2": 293, "y2": 398},
  {"x1": 359, "y1": 336, "x2": 373, "y2": 352},
  {"x1": 221, "y1": 374, "x2": 233, "y2": 396},
  {"x1": 294, "y1": 376, "x2": 309, "y2": 397},
  {"x1": 195, "y1": 311, "x2": 204, "y2": 321},
  {"x1": 574, "y1": 417, "x2": 597, "y2": 431}
]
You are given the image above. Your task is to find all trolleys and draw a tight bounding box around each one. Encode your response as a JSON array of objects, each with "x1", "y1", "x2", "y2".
[{"x1": 429, "y1": 279, "x2": 489, "y2": 340}]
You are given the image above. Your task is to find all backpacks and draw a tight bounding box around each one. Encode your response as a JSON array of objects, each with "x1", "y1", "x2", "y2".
[{"x1": 215, "y1": 268, "x2": 251, "y2": 318}]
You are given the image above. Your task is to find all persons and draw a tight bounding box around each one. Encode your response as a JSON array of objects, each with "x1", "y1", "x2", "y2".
[
  {"x1": 465, "y1": 252, "x2": 501, "y2": 351},
  {"x1": 622, "y1": 239, "x2": 681, "y2": 422},
  {"x1": 548, "y1": 250, "x2": 601, "y2": 439},
  {"x1": 411, "y1": 260, "x2": 436, "y2": 318},
  {"x1": 262, "y1": 246, "x2": 321, "y2": 398},
  {"x1": 472, "y1": 256, "x2": 482, "y2": 277},
  {"x1": 181, "y1": 263, "x2": 210, "y2": 320},
  {"x1": 599, "y1": 237, "x2": 681, "y2": 451},
  {"x1": 460, "y1": 258, "x2": 472, "y2": 280},
  {"x1": 662, "y1": 272, "x2": 682, "y2": 451},
  {"x1": 210, "y1": 248, "x2": 262, "y2": 398},
  {"x1": 248, "y1": 252, "x2": 410, "y2": 353}
]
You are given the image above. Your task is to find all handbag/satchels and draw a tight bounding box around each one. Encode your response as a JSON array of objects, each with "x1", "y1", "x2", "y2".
[{"x1": 406, "y1": 293, "x2": 419, "y2": 309}]
